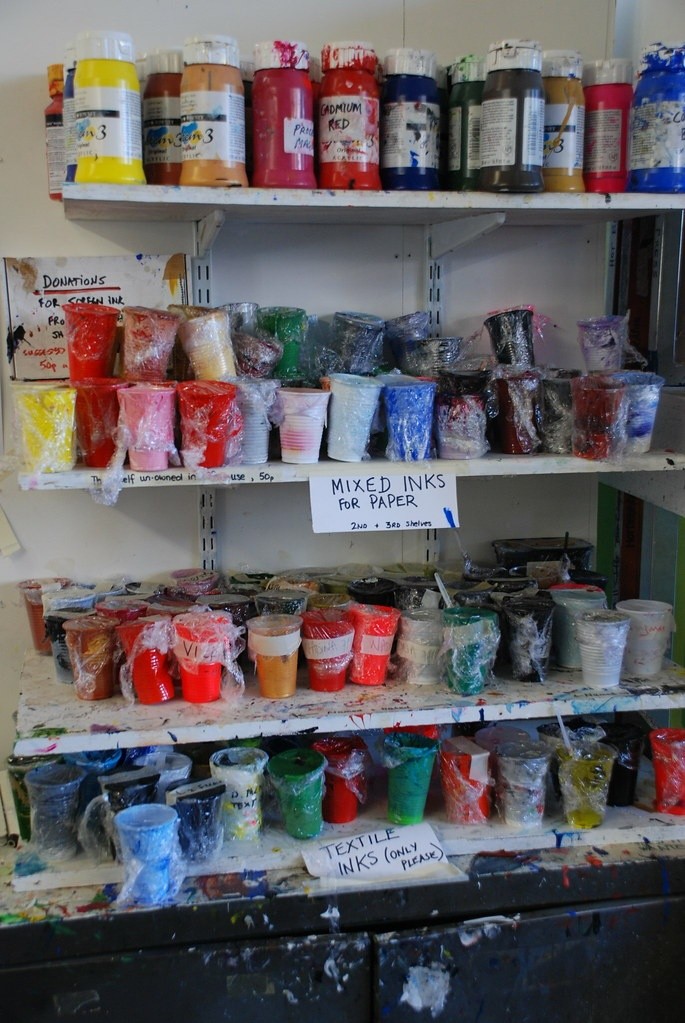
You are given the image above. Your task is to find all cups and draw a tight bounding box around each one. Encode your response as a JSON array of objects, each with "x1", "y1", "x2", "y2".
[
  {"x1": 5, "y1": 722, "x2": 685, "y2": 908},
  {"x1": 11, "y1": 304, "x2": 662, "y2": 476},
  {"x1": 19, "y1": 562, "x2": 672, "y2": 702}
]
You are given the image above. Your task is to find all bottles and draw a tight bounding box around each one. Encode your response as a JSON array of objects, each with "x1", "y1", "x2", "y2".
[{"x1": 42, "y1": 29, "x2": 685, "y2": 198}]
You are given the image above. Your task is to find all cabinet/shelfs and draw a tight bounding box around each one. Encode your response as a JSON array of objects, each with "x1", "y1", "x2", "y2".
[
  {"x1": 0, "y1": 894, "x2": 685, "y2": 1023},
  {"x1": 9, "y1": 182, "x2": 685, "y2": 894}
]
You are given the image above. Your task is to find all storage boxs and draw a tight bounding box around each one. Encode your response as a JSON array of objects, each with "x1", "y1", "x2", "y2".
[
  {"x1": 0, "y1": 253, "x2": 194, "y2": 380},
  {"x1": 491, "y1": 536, "x2": 596, "y2": 570}
]
